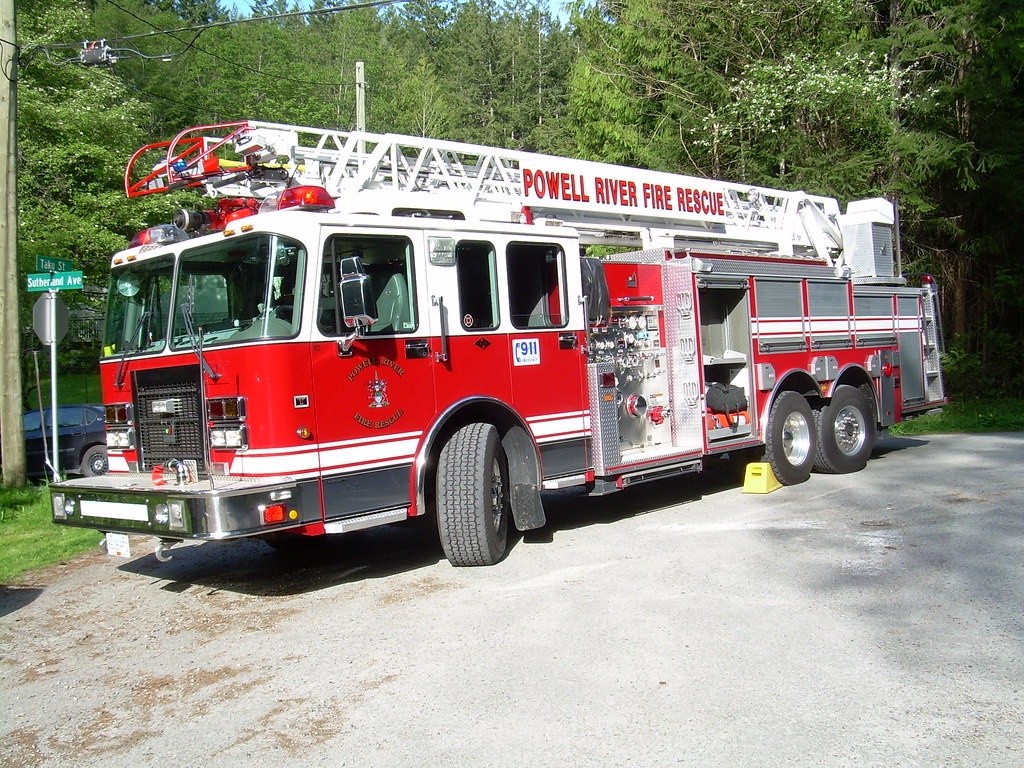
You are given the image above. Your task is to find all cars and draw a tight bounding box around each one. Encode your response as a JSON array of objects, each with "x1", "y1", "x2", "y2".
[{"x1": 23, "y1": 403, "x2": 107, "y2": 478}]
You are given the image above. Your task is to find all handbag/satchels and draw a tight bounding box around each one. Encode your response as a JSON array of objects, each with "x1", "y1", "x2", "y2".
[{"x1": 706, "y1": 383, "x2": 747, "y2": 428}]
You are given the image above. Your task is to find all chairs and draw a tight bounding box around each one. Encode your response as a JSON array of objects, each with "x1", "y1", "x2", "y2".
[{"x1": 352, "y1": 264, "x2": 410, "y2": 331}]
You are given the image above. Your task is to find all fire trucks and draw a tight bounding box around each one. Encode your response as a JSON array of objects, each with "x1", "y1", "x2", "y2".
[{"x1": 49, "y1": 121, "x2": 950, "y2": 568}]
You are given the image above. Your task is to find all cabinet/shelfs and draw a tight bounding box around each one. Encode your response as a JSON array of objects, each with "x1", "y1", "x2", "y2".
[{"x1": 698, "y1": 285, "x2": 752, "y2": 442}]
